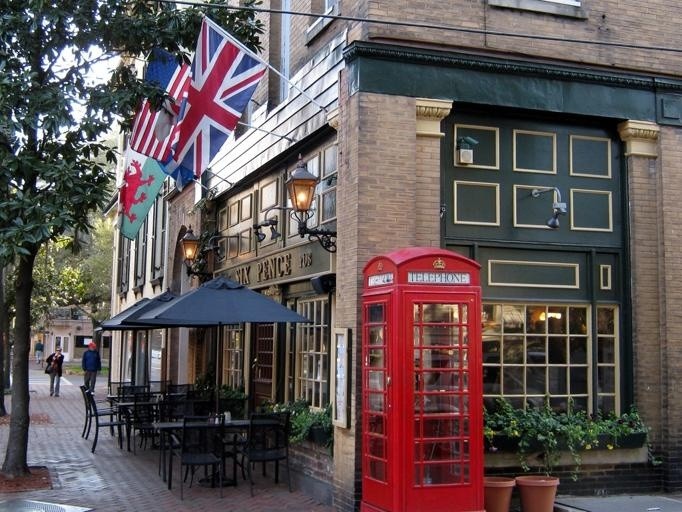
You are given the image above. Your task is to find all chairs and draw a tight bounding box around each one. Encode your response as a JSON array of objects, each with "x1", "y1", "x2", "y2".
[{"x1": 79, "y1": 380, "x2": 297, "y2": 500}]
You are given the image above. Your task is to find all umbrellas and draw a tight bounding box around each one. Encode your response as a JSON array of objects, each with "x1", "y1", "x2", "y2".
[
  {"x1": 120, "y1": 291, "x2": 180, "y2": 396},
  {"x1": 104, "y1": 298, "x2": 150, "y2": 384},
  {"x1": 155, "y1": 275, "x2": 312, "y2": 411}
]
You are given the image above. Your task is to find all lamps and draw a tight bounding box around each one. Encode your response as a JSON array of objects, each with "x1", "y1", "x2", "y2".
[
  {"x1": 533, "y1": 187, "x2": 570, "y2": 230},
  {"x1": 286, "y1": 156, "x2": 337, "y2": 255},
  {"x1": 175, "y1": 225, "x2": 224, "y2": 279}
]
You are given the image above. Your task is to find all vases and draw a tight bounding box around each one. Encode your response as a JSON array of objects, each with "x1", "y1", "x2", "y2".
[
  {"x1": 482, "y1": 475, "x2": 515, "y2": 511},
  {"x1": 516, "y1": 472, "x2": 560, "y2": 512}
]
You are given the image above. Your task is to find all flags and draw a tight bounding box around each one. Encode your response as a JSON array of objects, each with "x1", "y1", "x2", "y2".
[{"x1": 114, "y1": 16, "x2": 268, "y2": 240}]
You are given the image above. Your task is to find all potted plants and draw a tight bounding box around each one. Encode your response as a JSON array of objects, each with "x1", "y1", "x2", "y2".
[{"x1": 286, "y1": 405, "x2": 337, "y2": 453}]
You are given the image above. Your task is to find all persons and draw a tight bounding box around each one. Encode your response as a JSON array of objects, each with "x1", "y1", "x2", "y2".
[
  {"x1": 82, "y1": 342, "x2": 101, "y2": 396},
  {"x1": 45, "y1": 347, "x2": 64, "y2": 397},
  {"x1": 35, "y1": 339, "x2": 44, "y2": 364}
]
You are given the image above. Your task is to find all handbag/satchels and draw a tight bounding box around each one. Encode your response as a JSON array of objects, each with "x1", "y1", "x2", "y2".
[{"x1": 47, "y1": 366, "x2": 55, "y2": 374}]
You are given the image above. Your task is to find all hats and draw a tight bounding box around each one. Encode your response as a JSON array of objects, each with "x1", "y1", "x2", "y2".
[{"x1": 89, "y1": 342, "x2": 96, "y2": 349}]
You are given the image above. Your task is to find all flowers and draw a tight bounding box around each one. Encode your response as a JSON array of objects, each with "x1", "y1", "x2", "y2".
[
  {"x1": 456, "y1": 391, "x2": 561, "y2": 479},
  {"x1": 553, "y1": 397, "x2": 663, "y2": 484}
]
